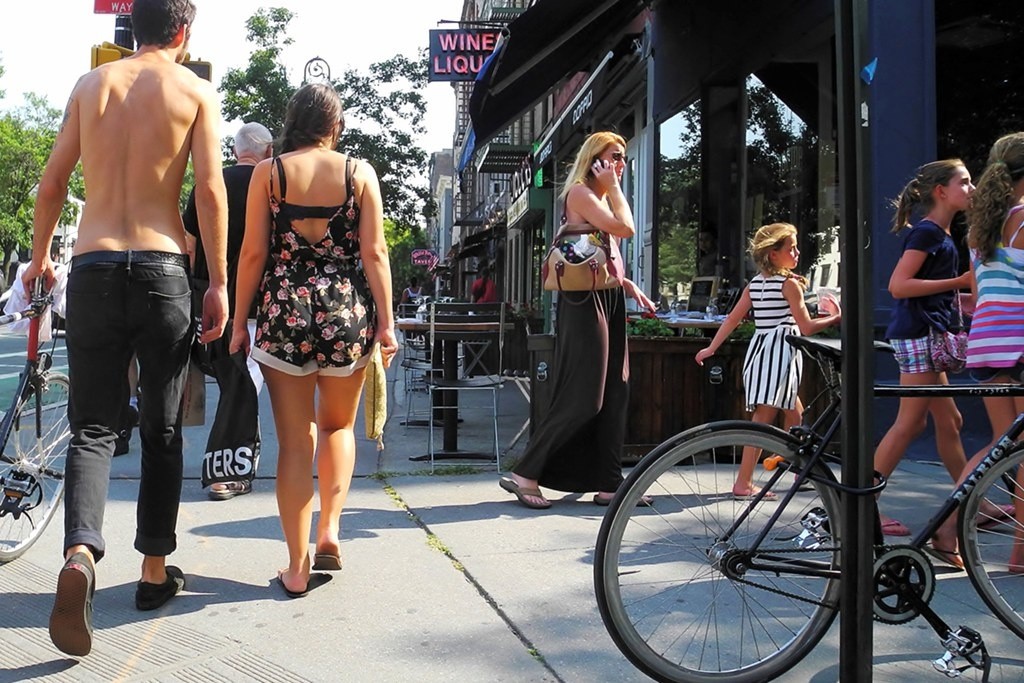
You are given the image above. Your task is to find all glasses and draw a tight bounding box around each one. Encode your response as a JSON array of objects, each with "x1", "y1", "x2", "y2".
[{"x1": 596, "y1": 151, "x2": 628, "y2": 162}]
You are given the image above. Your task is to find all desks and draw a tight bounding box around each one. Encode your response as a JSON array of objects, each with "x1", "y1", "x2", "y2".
[
  {"x1": 395, "y1": 322, "x2": 515, "y2": 461},
  {"x1": 630, "y1": 311, "x2": 730, "y2": 329}
]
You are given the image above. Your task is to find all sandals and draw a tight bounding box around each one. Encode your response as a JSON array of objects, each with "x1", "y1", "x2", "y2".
[{"x1": 208, "y1": 479, "x2": 251, "y2": 499}]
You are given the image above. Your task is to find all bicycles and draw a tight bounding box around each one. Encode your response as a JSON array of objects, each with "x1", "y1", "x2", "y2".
[
  {"x1": 0, "y1": 261, "x2": 74, "y2": 565},
  {"x1": 593, "y1": 334, "x2": 1024, "y2": 683}
]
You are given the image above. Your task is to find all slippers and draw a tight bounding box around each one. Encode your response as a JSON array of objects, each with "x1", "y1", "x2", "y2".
[
  {"x1": 923, "y1": 542, "x2": 965, "y2": 570},
  {"x1": 277, "y1": 567, "x2": 308, "y2": 597},
  {"x1": 312, "y1": 554, "x2": 344, "y2": 570},
  {"x1": 500, "y1": 476, "x2": 552, "y2": 509},
  {"x1": 594, "y1": 494, "x2": 655, "y2": 506},
  {"x1": 880, "y1": 519, "x2": 911, "y2": 535},
  {"x1": 797, "y1": 480, "x2": 816, "y2": 489},
  {"x1": 976, "y1": 504, "x2": 1017, "y2": 528},
  {"x1": 734, "y1": 487, "x2": 778, "y2": 500}
]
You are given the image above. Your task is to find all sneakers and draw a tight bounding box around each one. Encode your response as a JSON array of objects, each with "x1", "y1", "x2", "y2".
[
  {"x1": 136, "y1": 565, "x2": 185, "y2": 610},
  {"x1": 49, "y1": 552, "x2": 93, "y2": 656}
]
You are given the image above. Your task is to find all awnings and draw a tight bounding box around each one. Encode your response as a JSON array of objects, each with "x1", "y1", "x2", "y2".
[
  {"x1": 456, "y1": 0, "x2": 653, "y2": 170},
  {"x1": 456, "y1": 227, "x2": 495, "y2": 261}
]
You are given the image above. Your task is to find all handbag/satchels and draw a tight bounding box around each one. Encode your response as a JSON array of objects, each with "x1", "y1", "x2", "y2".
[
  {"x1": 181, "y1": 362, "x2": 206, "y2": 425},
  {"x1": 930, "y1": 328, "x2": 968, "y2": 373},
  {"x1": 541, "y1": 190, "x2": 625, "y2": 305},
  {"x1": 366, "y1": 342, "x2": 389, "y2": 451}
]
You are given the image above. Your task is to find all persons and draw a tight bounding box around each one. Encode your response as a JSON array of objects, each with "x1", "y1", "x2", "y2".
[
  {"x1": 181, "y1": 122, "x2": 274, "y2": 500},
  {"x1": 499, "y1": 131, "x2": 655, "y2": 508},
  {"x1": 471, "y1": 267, "x2": 496, "y2": 302},
  {"x1": 873, "y1": 160, "x2": 1015, "y2": 537},
  {"x1": 696, "y1": 228, "x2": 718, "y2": 294},
  {"x1": 921, "y1": 133, "x2": 1024, "y2": 574},
  {"x1": 114, "y1": 352, "x2": 138, "y2": 457},
  {"x1": 21, "y1": 0, "x2": 230, "y2": 656},
  {"x1": 696, "y1": 223, "x2": 841, "y2": 501},
  {"x1": 229, "y1": 84, "x2": 398, "y2": 598},
  {"x1": 401, "y1": 277, "x2": 423, "y2": 302}
]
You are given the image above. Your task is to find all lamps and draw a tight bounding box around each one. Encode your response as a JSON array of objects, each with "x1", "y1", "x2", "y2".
[{"x1": 483, "y1": 203, "x2": 502, "y2": 229}]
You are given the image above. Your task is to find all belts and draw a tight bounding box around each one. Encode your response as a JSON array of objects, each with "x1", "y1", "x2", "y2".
[{"x1": 71, "y1": 250, "x2": 187, "y2": 266}]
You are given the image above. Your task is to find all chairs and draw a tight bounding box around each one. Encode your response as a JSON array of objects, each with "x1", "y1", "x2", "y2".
[
  {"x1": 401, "y1": 304, "x2": 445, "y2": 426},
  {"x1": 430, "y1": 302, "x2": 506, "y2": 473}
]
